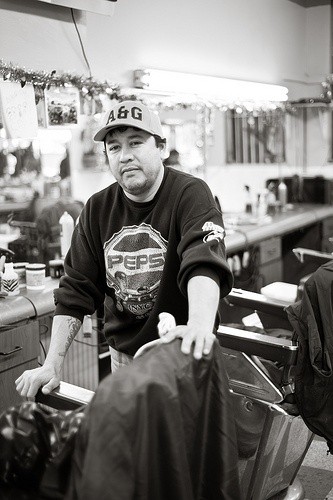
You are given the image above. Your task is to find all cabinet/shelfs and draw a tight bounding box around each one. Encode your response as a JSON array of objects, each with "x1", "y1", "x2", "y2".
[{"x1": 1, "y1": 277, "x2": 98, "y2": 428}]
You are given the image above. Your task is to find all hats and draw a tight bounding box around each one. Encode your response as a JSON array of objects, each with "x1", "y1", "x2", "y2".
[{"x1": 93, "y1": 99, "x2": 168, "y2": 141}]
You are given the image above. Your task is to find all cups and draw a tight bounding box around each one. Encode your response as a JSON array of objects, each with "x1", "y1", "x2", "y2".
[
  {"x1": 26, "y1": 264, "x2": 46, "y2": 290},
  {"x1": 49, "y1": 260, "x2": 65, "y2": 279},
  {"x1": 13, "y1": 263, "x2": 26, "y2": 288}
]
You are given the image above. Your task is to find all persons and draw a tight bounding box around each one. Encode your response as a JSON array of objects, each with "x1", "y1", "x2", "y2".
[
  {"x1": 163, "y1": 149, "x2": 180, "y2": 166},
  {"x1": 59, "y1": 142, "x2": 70, "y2": 181},
  {"x1": 13, "y1": 100, "x2": 235, "y2": 402}
]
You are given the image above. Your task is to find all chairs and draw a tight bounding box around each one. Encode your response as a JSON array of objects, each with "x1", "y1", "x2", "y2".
[
  {"x1": 0, "y1": 342, "x2": 240, "y2": 500},
  {"x1": 217, "y1": 258, "x2": 333, "y2": 495}
]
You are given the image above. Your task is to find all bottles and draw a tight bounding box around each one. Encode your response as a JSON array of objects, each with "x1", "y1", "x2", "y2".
[
  {"x1": 277, "y1": 182, "x2": 288, "y2": 208},
  {"x1": 59, "y1": 211, "x2": 74, "y2": 260}
]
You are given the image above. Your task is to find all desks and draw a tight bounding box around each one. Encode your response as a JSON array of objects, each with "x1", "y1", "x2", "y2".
[{"x1": 224, "y1": 199, "x2": 333, "y2": 286}]
